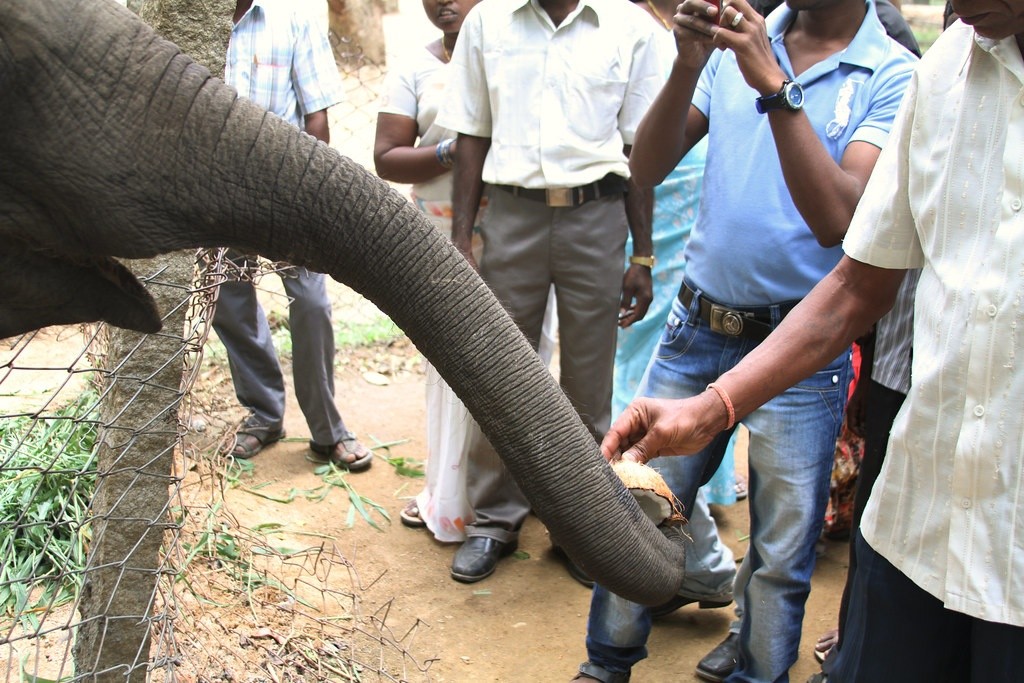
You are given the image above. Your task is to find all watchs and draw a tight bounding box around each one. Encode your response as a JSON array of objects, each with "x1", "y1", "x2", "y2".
[
  {"x1": 630, "y1": 255, "x2": 655, "y2": 268},
  {"x1": 756, "y1": 79, "x2": 805, "y2": 114}
]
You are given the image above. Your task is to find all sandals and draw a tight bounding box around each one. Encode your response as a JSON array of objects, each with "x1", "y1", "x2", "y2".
[{"x1": 568, "y1": 660, "x2": 632, "y2": 683}]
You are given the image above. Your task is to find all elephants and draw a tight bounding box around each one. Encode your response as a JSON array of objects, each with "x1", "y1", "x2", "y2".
[{"x1": 0, "y1": 0, "x2": 687, "y2": 606}]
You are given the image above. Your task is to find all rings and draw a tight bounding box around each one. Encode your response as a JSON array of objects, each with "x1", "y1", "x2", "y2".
[{"x1": 731, "y1": 12, "x2": 743, "y2": 28}]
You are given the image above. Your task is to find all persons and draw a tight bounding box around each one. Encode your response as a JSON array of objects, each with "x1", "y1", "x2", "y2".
[
  {"x1": 374, "y1": 0, "x2": 1024, "y2": 683},
  {"x1": 200, "y1": 0, "x2": 372, "y2": 468}
]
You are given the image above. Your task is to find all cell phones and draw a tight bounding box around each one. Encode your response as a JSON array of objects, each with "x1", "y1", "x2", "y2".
[{"x1": 701, "y1": 0, "x2": 723, "y2": 26}]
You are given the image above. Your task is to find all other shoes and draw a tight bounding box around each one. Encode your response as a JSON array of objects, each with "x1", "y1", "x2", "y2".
[
  {"x1": 694, "y1": 632, "x2": 744, "y2": 682},
  {"x1": 649, "y1": 594, "x2": 733, "y2": 613}
]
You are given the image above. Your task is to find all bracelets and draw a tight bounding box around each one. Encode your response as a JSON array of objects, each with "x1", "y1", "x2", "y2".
[
  {"x1": 436, "y1": 137, "x2": 456, "y2": 169},
  {"x1": 707, "y1": 383, "x2": 735, "y2": 430}
]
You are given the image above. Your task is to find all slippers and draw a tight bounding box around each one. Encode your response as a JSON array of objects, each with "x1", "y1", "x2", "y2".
[
  {"x1": 219, "y1": 420, "x2": 286, "y2": 459},
  {"x1": 734, "y1": 473, "x2": 748, "y2": 500},
  {"x1": 309, "y1": 432, "x2": 374, "y2": 470},
  {"x1": 399, "y1": 498, "x2": 427, "y2": 526},
  {"x1": 814, "y1": 628, "x2": 839, "y2": 665}
]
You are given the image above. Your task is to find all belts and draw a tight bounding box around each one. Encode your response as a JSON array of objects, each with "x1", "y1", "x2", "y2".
[
  {"x1": 500, "y1": 173, "x2": 624, "y2": 208},
  {"x1": 677, "y1": 279, "x2": 802, "y2": 337}
]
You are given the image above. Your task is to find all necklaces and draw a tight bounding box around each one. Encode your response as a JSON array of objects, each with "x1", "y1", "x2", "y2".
[
  {"x1": 648, "y1": 0, "x2": 671, "y2": 31},
  {"x1": 442, "y1": 35, "x2": 451, "y2": 62}
]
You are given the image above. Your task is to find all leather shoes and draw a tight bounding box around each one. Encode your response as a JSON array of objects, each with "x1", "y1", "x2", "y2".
[
  {"x1": 551, "y1": 545, "x2": 596, "y2": 587},
  {"x1": 449, "y1": 536, "x2": 519, "y2": 582}
]
